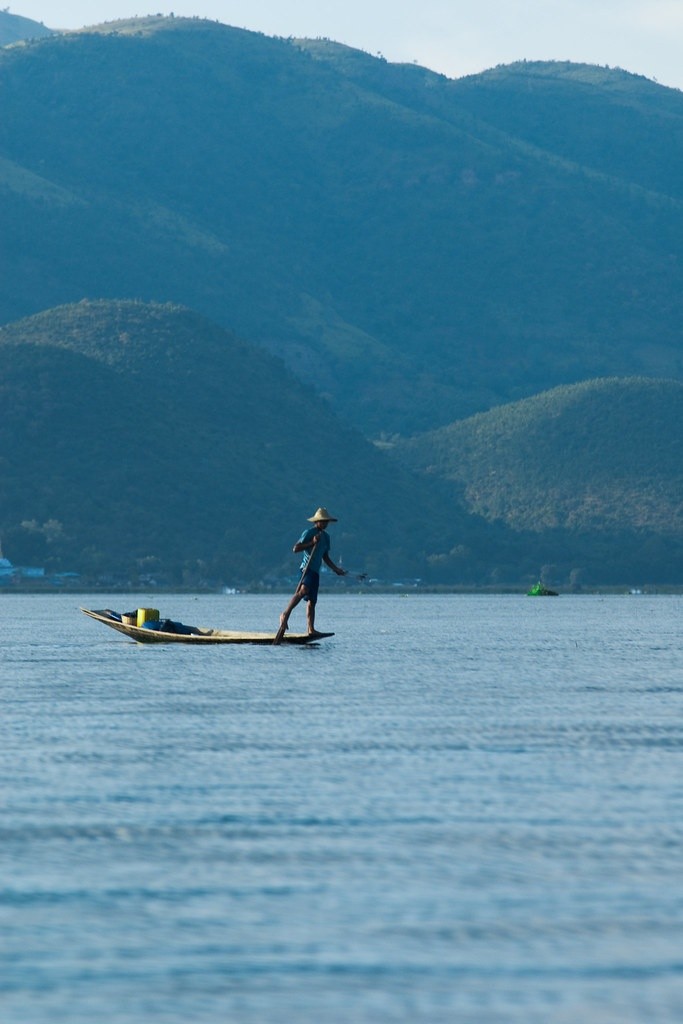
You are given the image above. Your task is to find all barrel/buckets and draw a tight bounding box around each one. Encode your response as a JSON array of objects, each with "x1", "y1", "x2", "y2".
[{"x1": 137, "y1": 608, "x2": 158, "y2": 626}]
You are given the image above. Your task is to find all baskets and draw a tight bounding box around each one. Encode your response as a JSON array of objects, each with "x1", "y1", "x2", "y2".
[{"x1": 122, "y1": 615, "x2": 137, "y2": 626}]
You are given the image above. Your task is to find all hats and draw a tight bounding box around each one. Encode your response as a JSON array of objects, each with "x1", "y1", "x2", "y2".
[{"x1": 307, "y1": 508, "x2": 338, "y2": 522}]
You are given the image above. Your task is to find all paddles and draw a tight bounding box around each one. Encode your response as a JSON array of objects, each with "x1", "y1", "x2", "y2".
[{"x1": 272, "y1": 543, "x2": 319, "y2": 645}]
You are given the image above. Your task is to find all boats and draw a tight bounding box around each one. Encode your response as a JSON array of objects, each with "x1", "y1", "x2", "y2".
[
  {"x1": 78, "y1": 605, "x2": 336, "y2": 644},
  {"x1": 526, "y1": 582, "x2": 558, "y2": 597}
]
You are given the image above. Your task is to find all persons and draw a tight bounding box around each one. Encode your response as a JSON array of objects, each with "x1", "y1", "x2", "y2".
[{"x1": 279, "y1": 508, "x2": 346, "y2": 634}]
